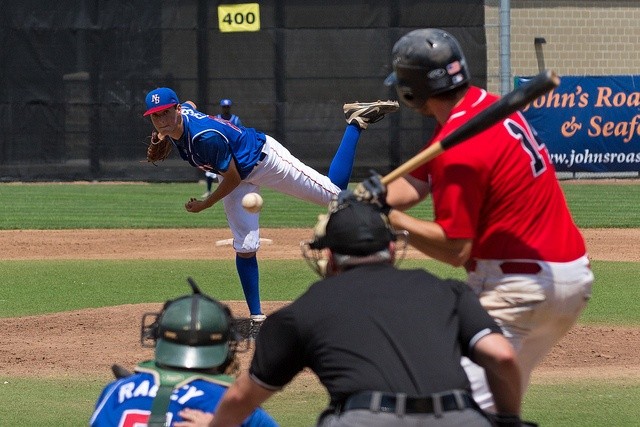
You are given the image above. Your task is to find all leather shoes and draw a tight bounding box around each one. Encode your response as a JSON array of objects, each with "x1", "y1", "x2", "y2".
[{"x1": 338, "y1": 390, "x2": 477, "y2": 416}]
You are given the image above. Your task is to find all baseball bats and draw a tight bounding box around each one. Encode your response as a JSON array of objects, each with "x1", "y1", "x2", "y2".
[{"x1": 363, "y1": 70, "x2": 560, "y2": 202}]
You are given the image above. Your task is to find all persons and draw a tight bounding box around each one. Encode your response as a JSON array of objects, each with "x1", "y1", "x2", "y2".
[
  {"x1": 347, "y1": 24, "x2": 596, "y2": 409},
  {"x1": 84, "y1": 292, "x2": 281, "y2": 426},
  {"x1": 201, "y1": 95, "x2": 245, "y2": 204},
  {"x1": 140, "y1": 85, "x2": 401, "y2": 334},
  {"x1": 170, "y1": 201, "x2": 541, "y2": 426}
]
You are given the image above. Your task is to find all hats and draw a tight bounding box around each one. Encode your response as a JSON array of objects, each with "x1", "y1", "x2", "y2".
[{"x1": 143, "y1": 85, "x2": 181, "y2": 117}]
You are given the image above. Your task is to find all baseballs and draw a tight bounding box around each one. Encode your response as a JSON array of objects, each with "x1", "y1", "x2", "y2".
[{"x1": 241, "y1": 193, "x2": 263, "y2": 213}]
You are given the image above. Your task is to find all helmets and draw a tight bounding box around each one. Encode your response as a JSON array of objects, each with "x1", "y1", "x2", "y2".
[
  {"x1": 384, "y1": 27, "x2": 472, "y2": 109},
  {"x1": 137, "y1": 277, "x2": 255, "y2": 370},
  {"x1": 308, "y1": 198, "x2": 411, "y2": 266}
]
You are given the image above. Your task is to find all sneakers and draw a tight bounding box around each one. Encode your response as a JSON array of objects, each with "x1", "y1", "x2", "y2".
[
  {"x1": 343, "y1": 99, "x2": 400, "y2": 129},
  {"x1": 250, "y1": 314, "x2": 268, "y2": 334}
]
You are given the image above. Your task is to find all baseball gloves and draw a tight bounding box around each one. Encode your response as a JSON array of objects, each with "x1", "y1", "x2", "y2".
[{"x1": 142, "y1": 131, "x2": 171, "y2": 167}]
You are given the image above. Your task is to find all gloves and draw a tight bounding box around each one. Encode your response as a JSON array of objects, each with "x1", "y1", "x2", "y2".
[
  {"x1": 356, "y1": 168, "x2": 393, "y2": 213},
  {"x1": 329, "y1": 187, "x2": 356, "y2": 213}
]
